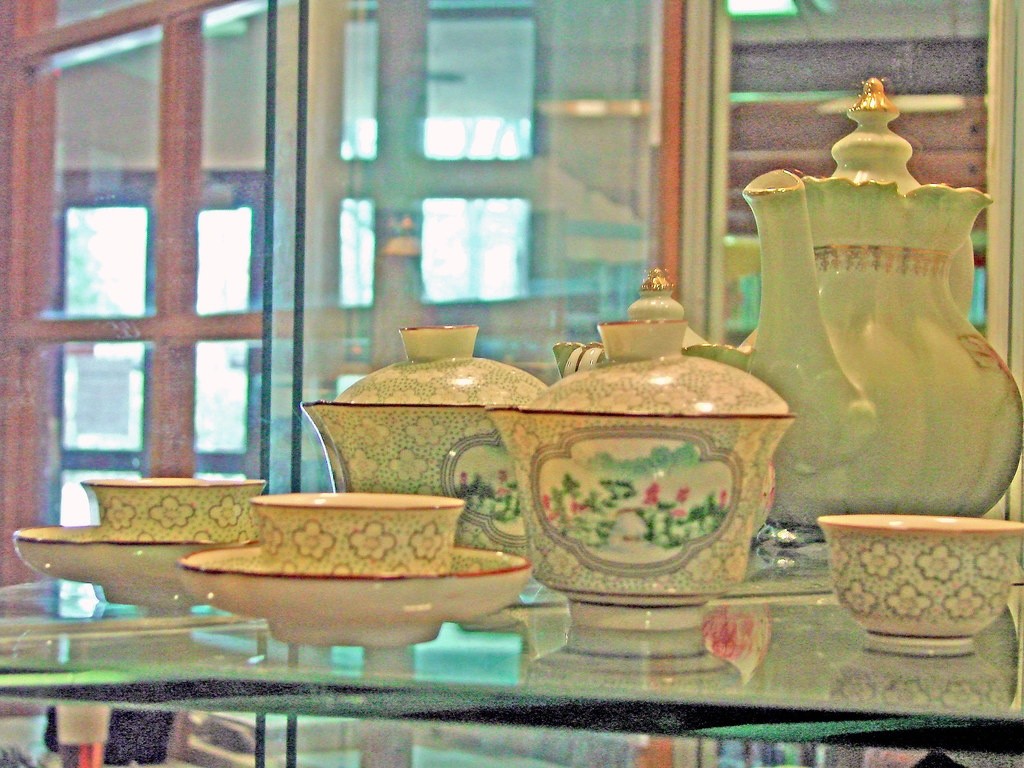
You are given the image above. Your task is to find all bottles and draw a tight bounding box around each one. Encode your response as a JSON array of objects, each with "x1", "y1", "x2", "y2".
[{"x1": 550, "y1": 342, "x2": 756, "y2": 382}]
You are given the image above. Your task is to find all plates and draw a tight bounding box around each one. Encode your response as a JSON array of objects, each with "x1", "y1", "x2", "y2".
[
  {"x1": 14, "y1": 526, "x2": 259, "y2": 608},
  {"x1": 180, "y1": 547, "x2": 529, "y2": 648}
]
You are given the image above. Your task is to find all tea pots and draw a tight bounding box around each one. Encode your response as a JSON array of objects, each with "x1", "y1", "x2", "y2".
[{"x1": 737, "y1": 76, "x2": 1024, "y2": 541}]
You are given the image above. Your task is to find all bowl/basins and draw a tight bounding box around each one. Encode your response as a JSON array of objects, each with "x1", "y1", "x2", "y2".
[{"x1": 818, "y1": 513, "x2": 1024, "y2": 661}]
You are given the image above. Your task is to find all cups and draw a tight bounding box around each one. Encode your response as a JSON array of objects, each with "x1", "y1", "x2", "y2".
[
  {"x1": 486, "y1": 315, "x2": 798, "y2": 635},
  {"x1": 80, "y1": 480, "x2": 262, "y2": 544},
  {"x1": 252, "y1": 492, "x2": 464, "y2": 575},
  {"x1": 301, "y1": 326, "x2": 551, "y2": 562}
]
